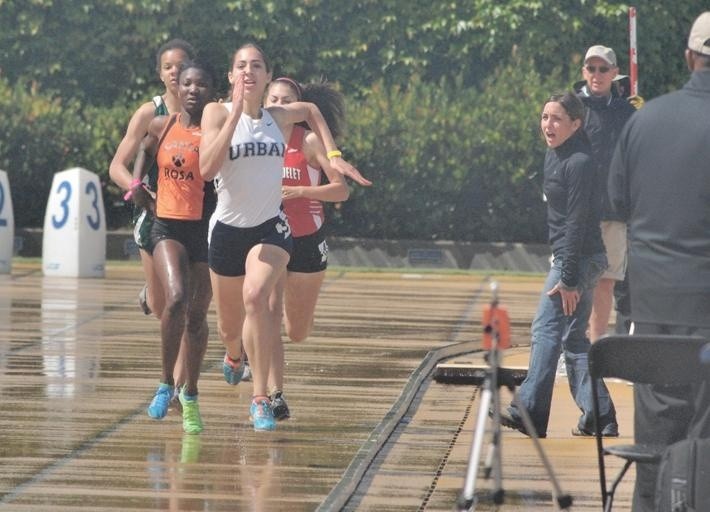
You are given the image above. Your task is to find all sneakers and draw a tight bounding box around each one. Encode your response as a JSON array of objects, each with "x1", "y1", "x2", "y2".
[
  {"x1": 572, "y1": 424, "x2": 619, "y2": 436},
  {"x1": 489, "y1": 407, "x2": 546, "y2": 437},
  {"x1": 148, "y1": 342, "x2": 291, "y2": 434}
]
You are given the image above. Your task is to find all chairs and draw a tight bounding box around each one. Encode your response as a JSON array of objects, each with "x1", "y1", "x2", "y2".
[{"x1": 587, "y1": 334, "x2": 710, "y2": 512}]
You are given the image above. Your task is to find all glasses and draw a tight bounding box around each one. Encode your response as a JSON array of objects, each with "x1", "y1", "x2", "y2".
[{"x1": 586, "y1": 66, "x2": 610, "y2": 74}]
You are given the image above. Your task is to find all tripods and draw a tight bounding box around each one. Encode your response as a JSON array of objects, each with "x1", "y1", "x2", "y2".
[{"x1": 456, "y1": 281, "x2": 572, "y2": 512}]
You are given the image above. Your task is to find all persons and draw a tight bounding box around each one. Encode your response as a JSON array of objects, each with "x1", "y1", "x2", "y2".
[
  {"x1": 607, "y1": 6, "x2": 710, "y2": 512},
  {"x1": 568, "y1": 44, "x2": 637, "y2": 346},
  {"x1": 610, "y1": 74, "x2": 632, "y2": 335},
  {"x1": 489, "y1": 90, "x2": 619, "y2": 439},
  {"x1": 108, "y1": 38, "x2": 372, "y2": 431}
]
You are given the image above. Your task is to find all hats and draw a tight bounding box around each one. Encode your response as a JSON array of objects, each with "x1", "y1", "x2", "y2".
[
  {"x1": 688, "y1": 10, "x2": 710, "y2": 55},
  {"x1": 584, "y1": 45, "x2": 617, "y2": 65}
]
